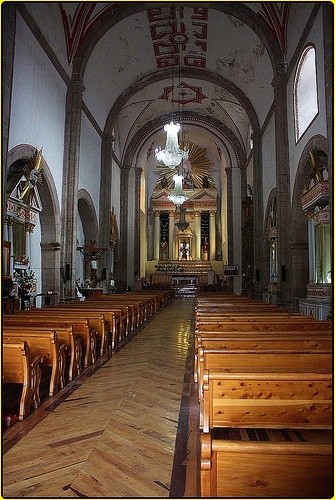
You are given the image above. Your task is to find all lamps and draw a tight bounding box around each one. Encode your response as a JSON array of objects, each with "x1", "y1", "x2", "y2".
[
  {"x1": 89, "y1": 260, "x2": 98, "y2": 270},
  {"x1": 109, "y1": 279, "x2": 114, "y2": 286},
  {"x1": 154, "y1": 1, "x2": 193, "y2": 206}
]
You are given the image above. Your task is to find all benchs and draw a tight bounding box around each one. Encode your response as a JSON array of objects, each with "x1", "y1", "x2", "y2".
[
  {"x1": 0, "y1": 285, "x2": 173, "y2": 421},
  {"x1": 194, "y1": 286, "x2": 334, "y2": 499}
]
